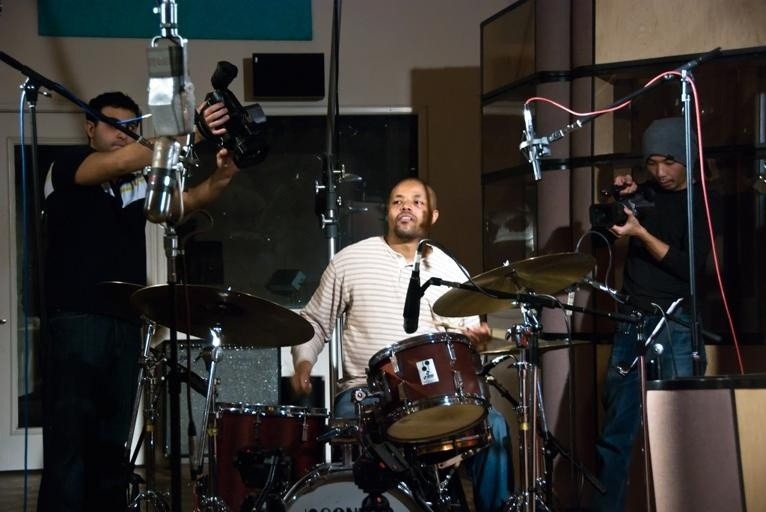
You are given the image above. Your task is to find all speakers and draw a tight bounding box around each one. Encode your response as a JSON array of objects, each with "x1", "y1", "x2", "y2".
[{"x1": 154, "y1": 339, "x2": 281, "y2": 457}]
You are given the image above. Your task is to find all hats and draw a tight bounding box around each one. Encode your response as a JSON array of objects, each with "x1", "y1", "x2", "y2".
[{"x1": 639, "y1": 115, "x2": 699, "y2": 173}]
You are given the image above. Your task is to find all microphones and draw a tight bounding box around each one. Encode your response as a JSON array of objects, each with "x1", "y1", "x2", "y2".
[
  {"x1": 523, "y1": 109, "x2": 544, "y2": 180},
  {"x1": 402, "y1": 267, "x2": 421, "y2": 333},
  {"x1": 147, "y1": 29, "x2": 194, "y2": 134},
  {"x1": 144, "y1": 137, "x2": 182, "y2": 224}
]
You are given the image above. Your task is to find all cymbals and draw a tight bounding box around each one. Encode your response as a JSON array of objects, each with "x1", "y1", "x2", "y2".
[
  {"x1": 474, "y1": 336, "x2": 593, "y2": 355},
  {"x1": 432, "y1": 252, "x2": 595, "y2": 317},
  {"x1": 130, "y1": 283, "x2": 314, "y2": 347}
]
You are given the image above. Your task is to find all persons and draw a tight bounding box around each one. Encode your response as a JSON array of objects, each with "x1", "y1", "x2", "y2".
[
  {"x1": 579, "y1": 116, "x2": 723, "y2": 512},
  {"x1": 288, "y1": 179, "x2": 515, "y2": 512},
  {"x1": 38, "y1": 92, "x2": 241, "y2": 512}
]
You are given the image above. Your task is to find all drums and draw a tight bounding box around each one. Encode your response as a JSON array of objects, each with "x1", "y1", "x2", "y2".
[
  {"x1": 206, "y1": 401, "x2": 328, "y2": 510},
  {"x1": 367, "y1": 333, "x2": 490, "y2": 449},
  {"x1": 271, "y1": 463, "x2": 435, "y2": 512},
  {"x1": 360, "y1": 403, "x2": 492, "y2": 452}
]
